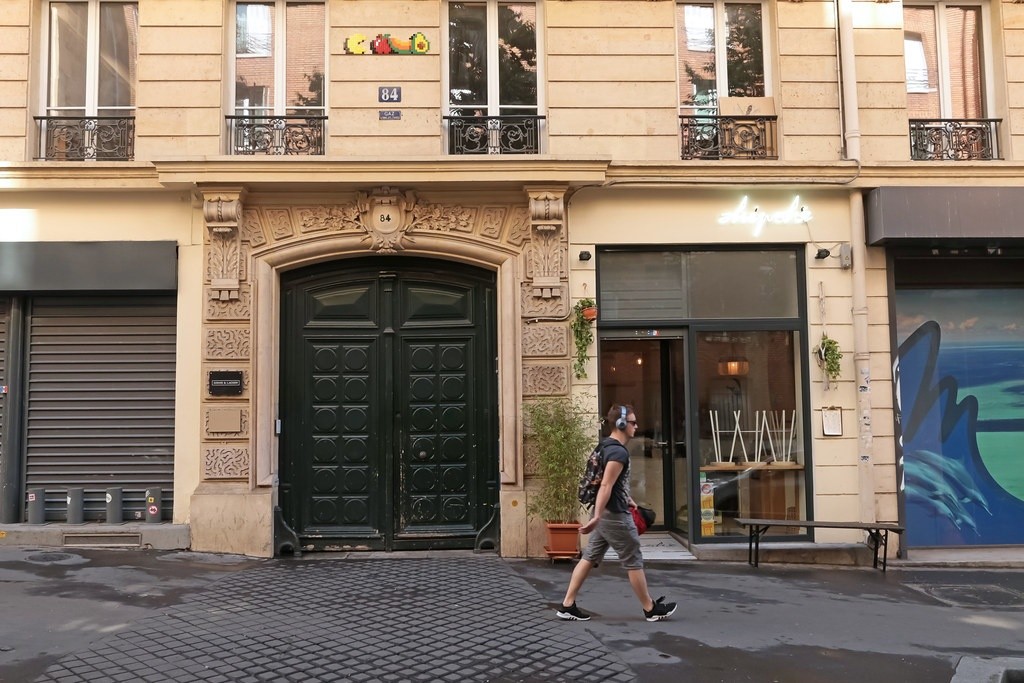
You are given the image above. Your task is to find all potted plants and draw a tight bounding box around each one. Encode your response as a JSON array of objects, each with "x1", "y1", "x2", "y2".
[
  {"x1": 570, "y1": 296, "x2": 597, "y2": 380},
  {"x1": 521, "y1": 385, "x2": 599, "y2": 564},
  {"x1": 812, "y1": 333, "x2": 842, "y2": 390}
]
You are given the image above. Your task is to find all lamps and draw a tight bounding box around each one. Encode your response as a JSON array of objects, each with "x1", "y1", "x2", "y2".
[{"x1": 717, "y1": 344, "x2": 749, "y2": 375}]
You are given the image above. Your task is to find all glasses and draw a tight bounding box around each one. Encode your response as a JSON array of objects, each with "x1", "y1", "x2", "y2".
[{"x1": 627, "y1": 420, "x2": 637, "y2": 426}]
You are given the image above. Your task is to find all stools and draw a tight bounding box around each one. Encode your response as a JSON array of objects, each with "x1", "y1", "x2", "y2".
[{"x1": 709, "y1": 408, "x2": 796, "y2": 465}]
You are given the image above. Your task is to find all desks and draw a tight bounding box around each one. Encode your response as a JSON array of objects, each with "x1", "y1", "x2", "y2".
[{"x1": 700, "y1": 464, "x2": 804, "y2": 531}]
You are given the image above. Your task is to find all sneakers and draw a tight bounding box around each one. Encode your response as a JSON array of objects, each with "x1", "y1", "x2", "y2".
[
  {"x1": 643, "y1": 595, "x2": 678, "y2": 622},
  {"x1": 556, "y1": 601, "x2": 591, "y2": 621}
]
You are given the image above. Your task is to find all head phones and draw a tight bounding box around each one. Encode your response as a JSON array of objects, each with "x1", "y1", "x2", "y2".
[{"x1": 616, "y1": 406, "x2": 627, "y2": 430}]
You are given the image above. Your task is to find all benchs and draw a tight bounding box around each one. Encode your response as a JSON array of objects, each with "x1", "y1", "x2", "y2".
[{"x1": 734, "y1": 517, "x2": 905, "y2": 571}]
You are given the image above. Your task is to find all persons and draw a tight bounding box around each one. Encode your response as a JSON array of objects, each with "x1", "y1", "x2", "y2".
[{"x1": 555, "y1": 404, "x2": 677, "y2": 622}]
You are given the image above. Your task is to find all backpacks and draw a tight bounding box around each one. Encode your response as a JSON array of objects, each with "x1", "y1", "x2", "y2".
[{"x1": 578, "y1": 442, "x2": 630, "y2": 505}]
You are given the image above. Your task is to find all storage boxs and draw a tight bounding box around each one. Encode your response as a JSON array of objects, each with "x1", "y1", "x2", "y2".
[{"x1": 699, "y1": 471, "x2": 722, "y2": 536}]
